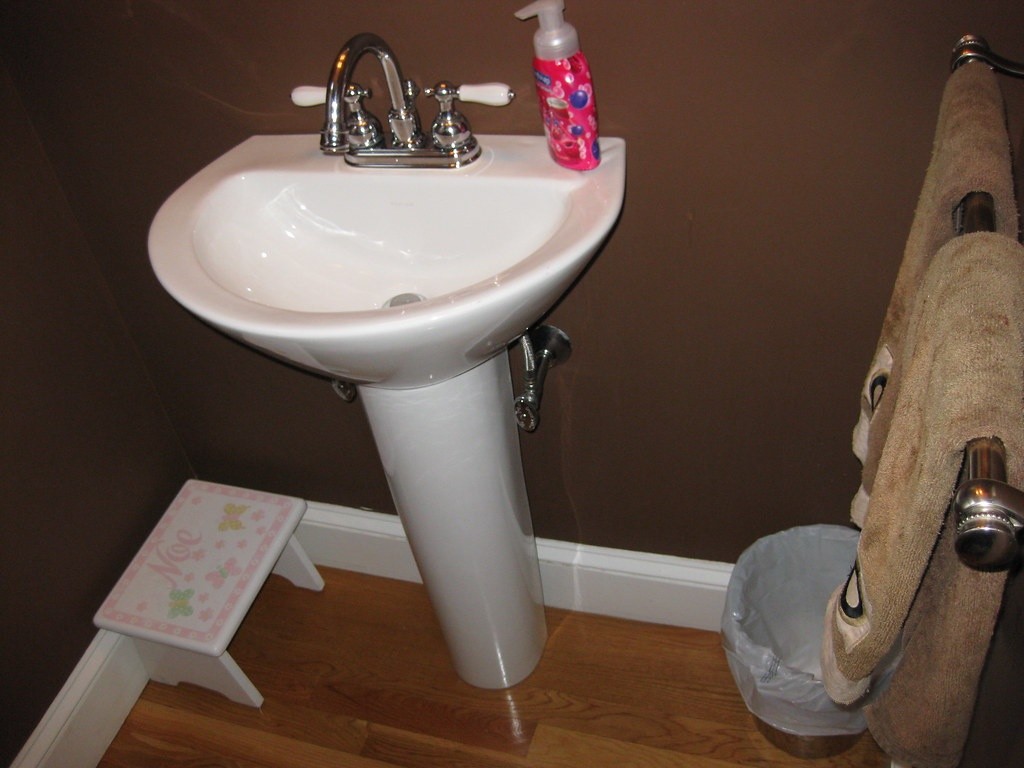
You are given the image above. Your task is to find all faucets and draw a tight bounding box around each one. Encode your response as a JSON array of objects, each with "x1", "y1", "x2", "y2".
[{"x1": 319, "y1": 31, "x2": 428, "y2": 156}]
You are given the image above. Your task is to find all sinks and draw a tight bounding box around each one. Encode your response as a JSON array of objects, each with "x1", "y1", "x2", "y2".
[{"x1": 146, "y1": 163, "x2": 625, "y2": 391}]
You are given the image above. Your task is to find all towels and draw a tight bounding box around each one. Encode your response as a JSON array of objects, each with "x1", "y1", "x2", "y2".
[{"x1": 816, "y1": 62, "x2": 1024, "y2": 768}]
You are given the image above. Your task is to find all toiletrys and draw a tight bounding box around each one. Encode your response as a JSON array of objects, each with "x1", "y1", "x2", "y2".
[{"x1": 514, "y1": 0, "x2": 602, "y2": 170}]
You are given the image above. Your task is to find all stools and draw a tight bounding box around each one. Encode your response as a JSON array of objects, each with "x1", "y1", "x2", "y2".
[{"x1": 91, "y1": 478, "x2": 325, "y2": 708}]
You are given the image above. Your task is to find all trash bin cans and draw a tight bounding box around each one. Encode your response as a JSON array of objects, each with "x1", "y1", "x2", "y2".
[{"x1": 725, "y1": 524, "x2": 903, "y2": 760}]
